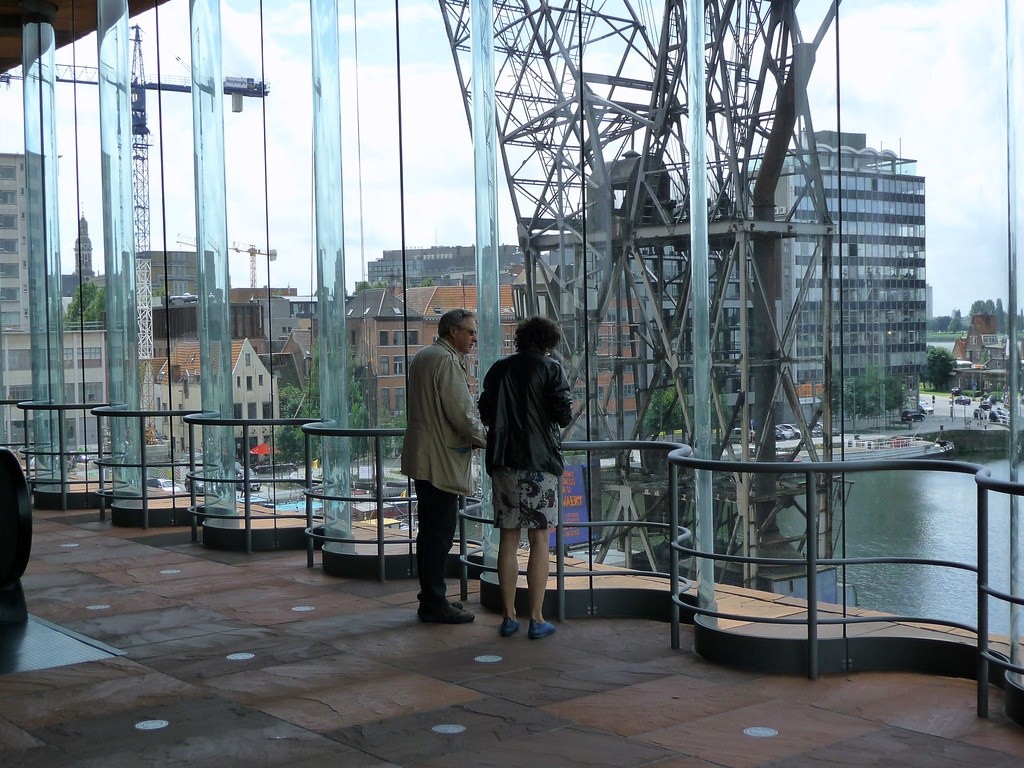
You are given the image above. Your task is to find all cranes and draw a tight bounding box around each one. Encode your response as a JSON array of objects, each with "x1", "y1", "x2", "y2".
[
  {"x1": 175, "y1": 231, "x2": 277, "y2": 288},
  {"x1": 0, "y1": 21, "x2": 271, "y2": 447}
]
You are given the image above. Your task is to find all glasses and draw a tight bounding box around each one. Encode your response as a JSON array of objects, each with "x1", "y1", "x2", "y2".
[{"x1": 456, "y1": 325, "x2": 477, "y2": 336}]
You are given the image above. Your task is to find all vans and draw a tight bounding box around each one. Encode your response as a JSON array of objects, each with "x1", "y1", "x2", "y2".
[{"x1": 235, "y1": 466, "x2": 261, "y2": 492}]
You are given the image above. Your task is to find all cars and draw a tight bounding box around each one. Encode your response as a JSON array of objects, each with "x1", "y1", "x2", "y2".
[
  {"x1": 723, "y1": 420, "x2": 841, "y2": 442},
  {"x1": 955, "y1": 396, "x2": 971, "y2": 407},
  {"x1": 146, "y1": 478, "x2": 180, "y2": 494},
  {"x1": 981, "y1": 394, "x2": 997, "y2": 405},
  {"x1": 951, "y1": 387, "x2": 961, "y2": 393},
  {"x1": 918, "y1": 401, "x2": 933, "y2": 414},
  {"x1": 901, "y1": 411, "x2": 926, "y2": 423},
  {"x1": 185, "y1": 475, "x2": 207, "y2": 493}
]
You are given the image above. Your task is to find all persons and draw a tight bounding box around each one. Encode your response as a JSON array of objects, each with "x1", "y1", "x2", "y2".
[
  {"x1": 478, "y1": 315, "x2": 573, "y2": 639},
  {"x1": 399, "y1": 308, "x2": 488, "y2": 624}
]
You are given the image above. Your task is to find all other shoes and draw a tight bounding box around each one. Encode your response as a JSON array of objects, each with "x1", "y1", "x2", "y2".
[
  {"x1": 446, "y1": 599, "x2": 464, "y2": 609},
  {"x1": 500, "y1": 616, "x2": 520, "y2": 636},
  {"x1": 418, "y1": 607, "x2": 475, "y2": 624},
  {"x1": 528, "y1": 619, "x2": 555, "y2": 639}
]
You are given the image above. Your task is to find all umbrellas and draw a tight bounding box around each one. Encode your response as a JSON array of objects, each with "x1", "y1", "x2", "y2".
[{"x1": 249, "y1": 444, "x2": 279, "y2": 456}]
